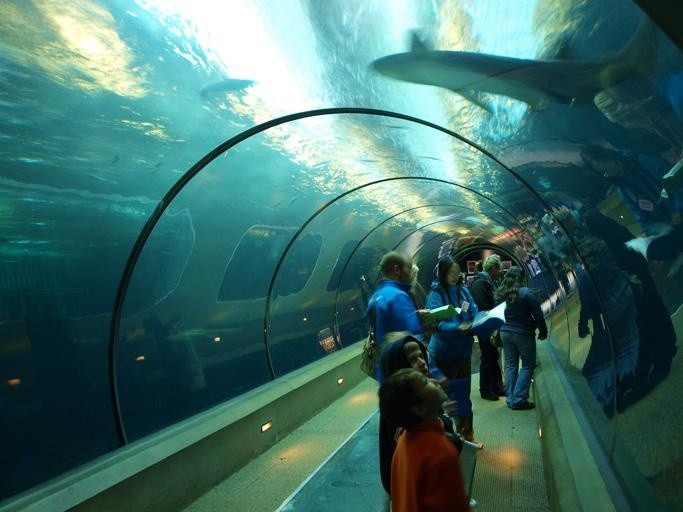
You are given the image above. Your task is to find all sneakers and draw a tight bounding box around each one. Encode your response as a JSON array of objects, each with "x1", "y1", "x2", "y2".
[
  {"x1": 507, "y1": 400, "x2": 535, "y2": 410},
  {"x1": 480, "y1": 390, "x2": 505, "y2": 401}
]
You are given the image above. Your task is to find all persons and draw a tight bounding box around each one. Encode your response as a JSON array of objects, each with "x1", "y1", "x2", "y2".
[
  {"x1": 378, "y1": 369, "x2": 468, "y2": 512},
  {"x1": 542, "y1": 64, "x2": 681, "y2": 420},
  {"x1": 362, "y1": 252, "x2": 479, "y2": 443},
  {"x1": 471, "y1": 254, "x2": 548, "y2": 410},
  {"x1": 370, "y1": 331, "x2": 483, "y2": 505}
]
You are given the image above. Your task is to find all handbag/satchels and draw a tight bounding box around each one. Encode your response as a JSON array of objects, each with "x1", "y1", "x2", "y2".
[
  {"x1": 361, "y1": 333, "x2": 380, "y2": 379},
  {"x1": 490, "y1": 329, "x2": 503, "y2": 348}
]
